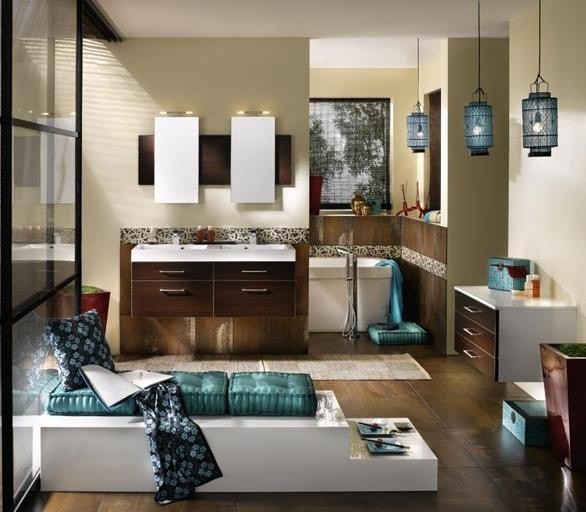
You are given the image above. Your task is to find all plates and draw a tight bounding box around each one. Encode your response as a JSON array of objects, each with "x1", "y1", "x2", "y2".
[{"x1": 354, "y1": 422, "x2": 413, "y2": 454}]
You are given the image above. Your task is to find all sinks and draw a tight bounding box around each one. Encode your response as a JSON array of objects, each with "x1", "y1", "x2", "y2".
[
  {"x1": 130, "y1": 244, "x2": 208, "y2": 263},
  {"x1": 219, "y1": 244, "x2": 297, "y2": 263}
]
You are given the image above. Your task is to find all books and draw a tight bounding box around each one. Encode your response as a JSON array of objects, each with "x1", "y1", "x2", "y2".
[{"x1": 78, "y1": 363, "x2": 175, "y2": 413}]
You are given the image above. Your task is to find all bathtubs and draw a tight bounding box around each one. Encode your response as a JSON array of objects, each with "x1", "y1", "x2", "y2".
[{"x1": 308, "y1": 257, "x2": 393, "y2": 333}]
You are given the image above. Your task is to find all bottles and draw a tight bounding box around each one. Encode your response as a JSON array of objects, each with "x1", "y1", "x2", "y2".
[{"x1": 524, "y1": 274, "x2": 540, "y2": 299}]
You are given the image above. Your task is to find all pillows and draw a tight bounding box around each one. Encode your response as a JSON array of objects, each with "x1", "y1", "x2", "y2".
[
  {"x1": 12, "y1": 310, "x2": 49, "y2": 390},
  {"x1": 42, "y1": 308, "x2": 115, "y2": 392}
]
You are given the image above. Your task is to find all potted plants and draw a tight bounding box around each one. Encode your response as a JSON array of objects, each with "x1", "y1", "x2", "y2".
[
  {"x1": 53, "y1": 284, "x2": 76, "y2": 318},
  {"x1": 81, "y1": 285, "x2": 110, "y2": 338},
  {"x1": 539, "y1": 342, "x2": 586, "y2": 471}
]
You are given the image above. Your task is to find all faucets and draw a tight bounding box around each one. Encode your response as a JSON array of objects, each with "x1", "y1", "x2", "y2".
[
  {"x1": 172, "y1": 230, "x2": 179, "y2": 244},
  {"x1": 337, "y1": 249, "x2": 356, "y2": 258},
  {"x1": 248, "y1": 229, "x2": 256, "y2": 244}
]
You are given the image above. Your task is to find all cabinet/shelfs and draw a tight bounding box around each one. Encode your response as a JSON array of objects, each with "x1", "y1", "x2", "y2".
[
  {"x1": 11, "y1": 260, "x2": 77, "y2": 320},
  {"x1": 130, "y1": 262, "x2": 296, "y2": 318},
  {"x1": 453, "y1": 284, "x2": 577, "y2": 385}
]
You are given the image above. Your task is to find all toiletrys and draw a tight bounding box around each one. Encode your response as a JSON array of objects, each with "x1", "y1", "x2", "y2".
[
  {"x1": 207, "y1": 225, "x2": 215, "y2": 243},
  {"x1": 196, "y1": 226, "x2": 203, "y2": 244},
  {"x1": 524, "y1": 274, "x2": 540, "y2": 299}
]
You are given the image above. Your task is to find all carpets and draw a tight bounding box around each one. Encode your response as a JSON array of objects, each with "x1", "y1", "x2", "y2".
[{"x1": 113, "y1": 351, "x2": 433, "y2": 381}]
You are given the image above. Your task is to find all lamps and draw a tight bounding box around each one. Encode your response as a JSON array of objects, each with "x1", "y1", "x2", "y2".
[{"x1": 405, "y1": 0, "x2": 558, "y2": 158}]
